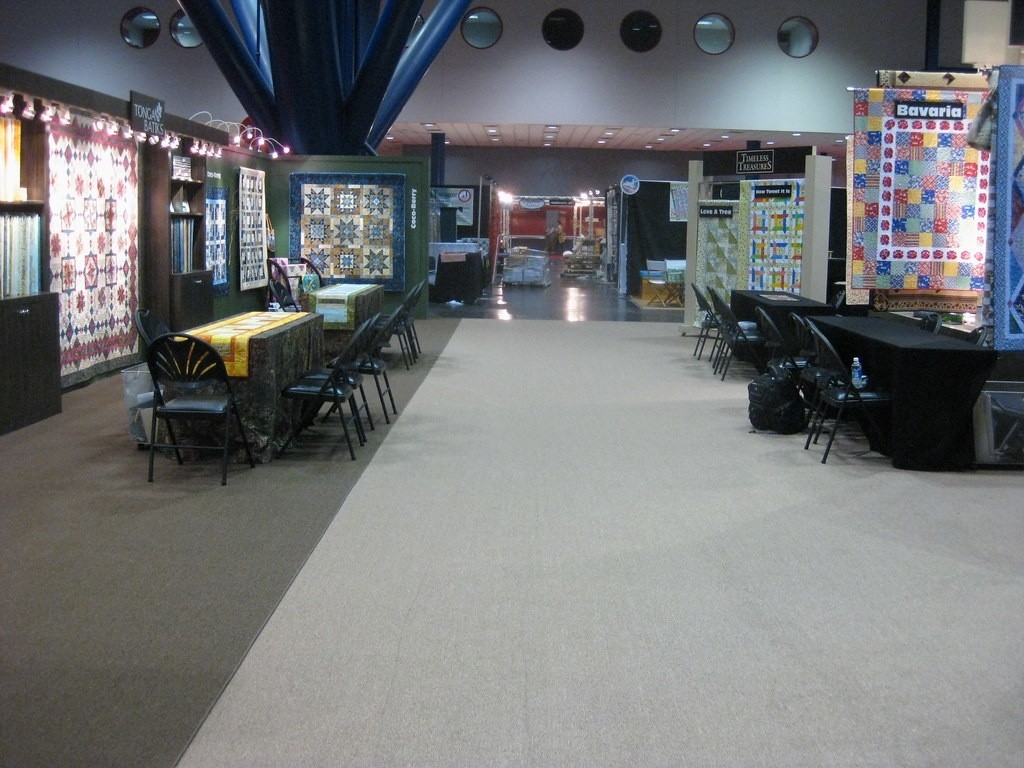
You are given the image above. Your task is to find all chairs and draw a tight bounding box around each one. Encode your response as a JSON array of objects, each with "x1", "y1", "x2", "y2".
[
  {"x1": 689, "y1": 284, "x2": 989, "y2": 463},
  {"x1": 646, "y1": 259, "x2": 686, "y2": 308},
  {"x1": 136, "y1": 278, "x2": 428, "y2": 487}
]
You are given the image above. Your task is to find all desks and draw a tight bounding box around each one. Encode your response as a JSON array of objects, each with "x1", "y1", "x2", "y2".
[
  {"x1": 434, "y1": 250, "x2": 483, "y2": 303},
  {"x1": 299, "y1": 283, "x2": 386, "y2": 368},
  {"x1": 805, "y1": 315, "x2": 1000, "y2": 472},
  {"x1": 152, "y1": 311, "x2": 326, "y2": 464},
  {"x1": 728, "y1": 290, "x2": 833, "y2": 358}
]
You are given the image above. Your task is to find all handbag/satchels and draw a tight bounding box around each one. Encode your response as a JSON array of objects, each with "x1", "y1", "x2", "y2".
[{"x1": 748, "y1": 373, "x2": 806, "y2": 434}]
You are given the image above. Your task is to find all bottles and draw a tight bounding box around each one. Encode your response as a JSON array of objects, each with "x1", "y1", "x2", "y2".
[{"x1": 851, "y1": 358, "x2": 862, "y2": 389}]
